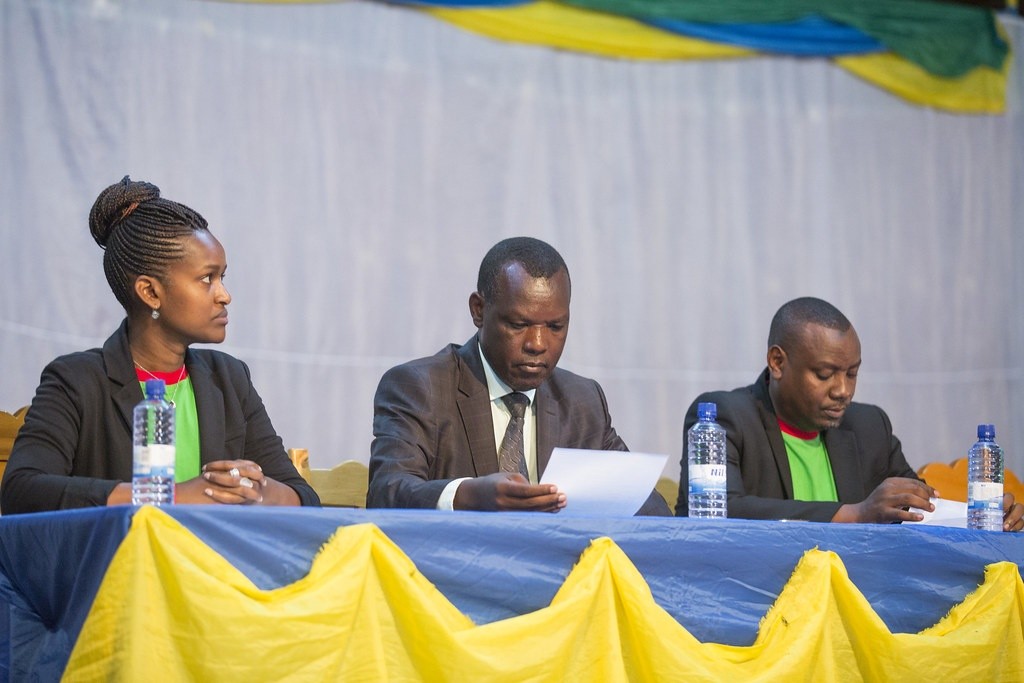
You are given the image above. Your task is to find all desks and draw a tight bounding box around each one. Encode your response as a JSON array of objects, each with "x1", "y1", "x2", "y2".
[{"x1": 2, "y1": 502, "x2": 1024, "y2": 681}]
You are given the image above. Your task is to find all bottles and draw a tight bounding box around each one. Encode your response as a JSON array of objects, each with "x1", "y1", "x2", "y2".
[
  {"x1": 133, "y1": 379, "x2": 176, "y2": 505},
  {"x1": 967, "y1": 423, "x2": 1003, "y2": 532},
  {"x1": 688, "y1": 403, "x2": 728, "y2": 519}
]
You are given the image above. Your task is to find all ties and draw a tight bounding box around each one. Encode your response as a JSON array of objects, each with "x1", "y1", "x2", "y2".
[{"x1": 499, "y1": 392, "x2": 530, "y2": 479}]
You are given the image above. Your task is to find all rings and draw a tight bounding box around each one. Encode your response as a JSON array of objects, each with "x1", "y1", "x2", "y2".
[
  {"x1": 229, "y1": 467, "x2": 239, "y2": 477},
  {"x1": 239, "y1": 476, "x2": 253, "y2": 488}
]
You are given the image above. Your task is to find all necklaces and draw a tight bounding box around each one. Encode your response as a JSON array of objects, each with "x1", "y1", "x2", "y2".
[{"x1": 133, "y1": 360, "x2": 185, "y2": 408}]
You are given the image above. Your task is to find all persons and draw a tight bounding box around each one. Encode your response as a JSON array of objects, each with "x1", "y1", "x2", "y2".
[
  {"x1": 365, "y1": 236, "x2": 673, "y2": 517},
  {"x1": 0, "y1": 175, "x2": 320, "y2": 514},
  {"x1": 673, "y1": 295, "x2": 1023, "y2": 531}
]
